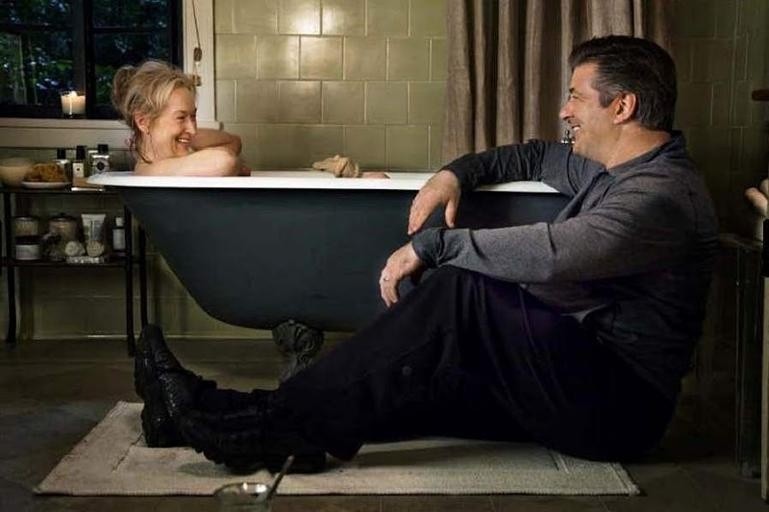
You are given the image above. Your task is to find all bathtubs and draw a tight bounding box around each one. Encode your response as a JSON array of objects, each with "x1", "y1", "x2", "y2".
[{"x1": 87, "y1": 166, "x2": 574, "y2": 386}]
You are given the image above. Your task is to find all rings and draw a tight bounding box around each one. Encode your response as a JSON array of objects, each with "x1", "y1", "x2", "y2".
[{"x1": 383, "y1": 277, "x2": 391, "y2": 282}]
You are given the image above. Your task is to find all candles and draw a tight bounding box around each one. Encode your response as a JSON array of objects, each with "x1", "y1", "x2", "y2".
[{"x1": 60, "y1": 94, "x2": 87, "y2": 115}]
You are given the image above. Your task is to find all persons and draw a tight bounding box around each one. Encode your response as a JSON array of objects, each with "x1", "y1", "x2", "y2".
[
  {"x1": 133, "y1": 34, "x2": 722, "y2": 474},
  {"x1": 109, "y1": 59, "x2": 390, "y2": 177}
]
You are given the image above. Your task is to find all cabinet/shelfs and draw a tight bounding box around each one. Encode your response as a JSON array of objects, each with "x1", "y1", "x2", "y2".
[{"x1": 0, "y1": 186, "x2": 149, "y2": 358}]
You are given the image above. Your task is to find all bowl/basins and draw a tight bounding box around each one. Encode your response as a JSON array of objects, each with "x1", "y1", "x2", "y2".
[{"x1": 0, "y1": 157, "x2": 35, "y2": 188}]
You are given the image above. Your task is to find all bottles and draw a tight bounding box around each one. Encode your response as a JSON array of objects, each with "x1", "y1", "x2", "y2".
[
  {"x1": 55, "y1": 143, "x2": 111, "y2": 186},
  {"x1": 11, "y1": 214, "x2": 77, "y2": 256},
  {"x1": 112, "y1": 217, "x2": 126, "y2": 252}
]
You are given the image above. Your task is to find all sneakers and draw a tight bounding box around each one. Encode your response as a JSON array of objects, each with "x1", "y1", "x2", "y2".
[
  {"x1": 181, "y1": 396, "x2": 326, "y2": 474},
  {"x1": 134, "y1": 323, "x2": 215, "y2": 447}
]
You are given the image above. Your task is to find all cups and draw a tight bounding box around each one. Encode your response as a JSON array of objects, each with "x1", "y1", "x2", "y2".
[
  {"x1": 212, "y1": 480, "x2": 270, "y2": 510},
  {"x1": 82, "y1": 213, "x2": 105, "y2": 243}
]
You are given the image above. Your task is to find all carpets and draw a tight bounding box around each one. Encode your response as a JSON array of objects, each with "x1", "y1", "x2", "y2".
[{"x1": 30, "y1": 399, "x2": 641, "y2": 495}]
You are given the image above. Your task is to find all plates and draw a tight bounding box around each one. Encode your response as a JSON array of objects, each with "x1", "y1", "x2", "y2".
[{"x1": 22, "y1": 180, "x2": 70, "y2": 189}]
